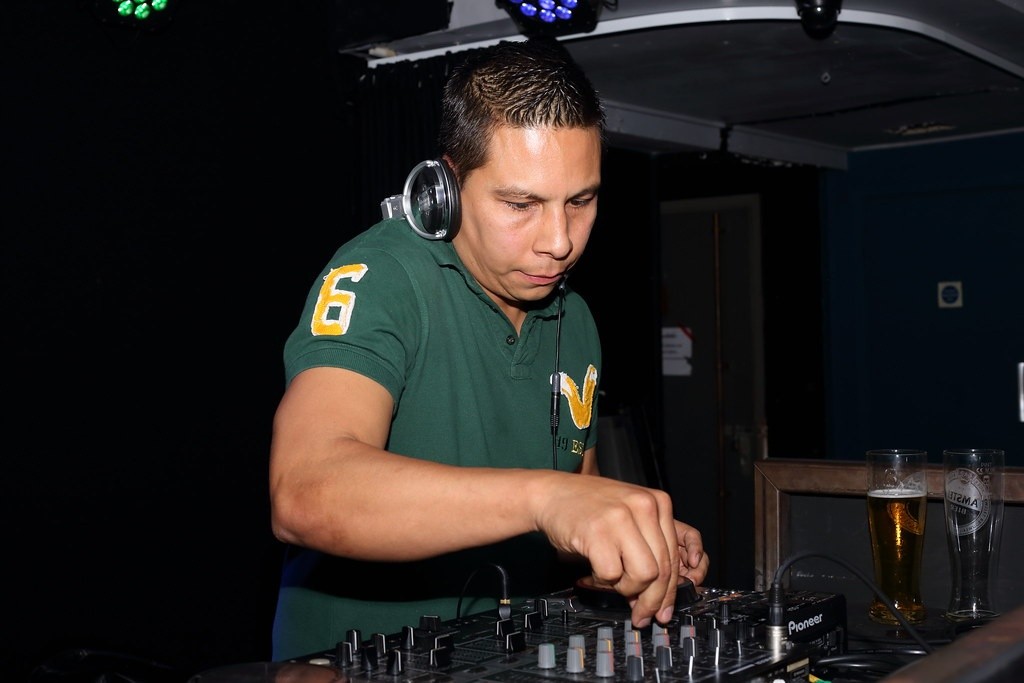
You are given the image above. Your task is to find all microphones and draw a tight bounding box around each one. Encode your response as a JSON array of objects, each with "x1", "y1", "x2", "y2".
[{"x1": 381, "y1": 156, "x2": 462, "y2": 242}]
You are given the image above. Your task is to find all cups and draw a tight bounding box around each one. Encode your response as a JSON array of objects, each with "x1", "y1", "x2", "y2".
[
  {"x1": 864, "y1": 448, "x2": 930, "y2": 626},
  {"x1": 942, "y1": 446, "x2": 1002, "y2": 623}
]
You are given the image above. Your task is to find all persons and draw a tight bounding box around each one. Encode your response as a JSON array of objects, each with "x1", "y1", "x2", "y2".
[{"x1": 268, "y1": 48, "x2": 711, "y2": 665}]
